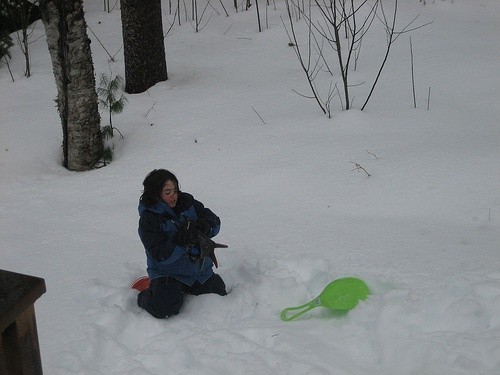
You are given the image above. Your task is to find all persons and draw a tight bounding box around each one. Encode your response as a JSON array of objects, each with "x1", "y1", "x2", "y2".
[{"x1": 130, "y1": 167, "x2": 228, "y2": 320}]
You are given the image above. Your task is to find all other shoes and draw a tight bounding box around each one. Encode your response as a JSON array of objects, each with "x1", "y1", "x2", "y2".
[{"x1": 132, "y1": 275, "x2": 150, "y2": 291}]
